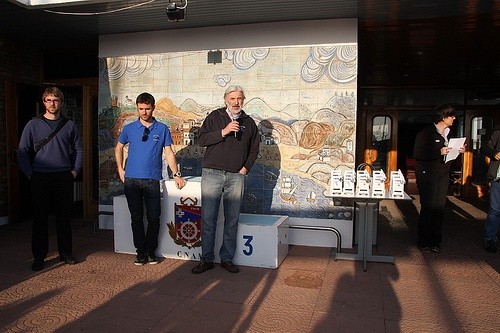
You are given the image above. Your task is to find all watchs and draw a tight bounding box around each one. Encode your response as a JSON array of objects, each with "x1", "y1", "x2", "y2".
[{"x1": 173, "y1": 172, "x2": 181, "y2": 177}]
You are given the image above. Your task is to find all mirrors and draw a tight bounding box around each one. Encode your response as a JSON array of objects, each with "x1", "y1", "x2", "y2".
[
  {"x1": 372, "y1": 115, "x2": 392, "y2": 171},
  {"x1": 471, "y1": 116, "x2": 493, "y2": 157}
]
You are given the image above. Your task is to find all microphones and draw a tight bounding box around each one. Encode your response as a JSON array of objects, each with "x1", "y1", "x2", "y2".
[{"x1": 232, "y1": 114, "x2": 240, "y2": 137}]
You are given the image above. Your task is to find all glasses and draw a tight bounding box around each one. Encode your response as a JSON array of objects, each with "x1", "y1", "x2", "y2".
[
  {"x1": 44, "y1": 99, "x2": 61, "y2": 104},
  {"x1": 142, "y1": 127, "x2": 148, "y2": 142}
]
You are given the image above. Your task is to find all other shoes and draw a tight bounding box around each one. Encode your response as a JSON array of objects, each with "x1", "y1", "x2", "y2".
[
  {"x1": 485, "y1": 247, "x2": 497, "y2": 252},
  {"x1": 31, "y1": 261, "x2": 43, "y2": 271},
  {"x1": 420, "y1": 245, "x2": 439, "y2": 253},
  {"x1": 60, "y1": 254, "x2": 77, "y2": 265}
]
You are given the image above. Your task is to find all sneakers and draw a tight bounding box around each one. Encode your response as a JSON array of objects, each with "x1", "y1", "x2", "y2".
[
  {"x1": 146, "y1": 251, "x2": 158, "y2": 264},
  {"x1": 134, "y1": 254, "x2": 147, "y2": 266},
  {"x1": 222, "y1": 261, "x2": 240, "y2": 273},
  {"x1": 192, "y1": 263, "x2": 212, "y2": 273}
]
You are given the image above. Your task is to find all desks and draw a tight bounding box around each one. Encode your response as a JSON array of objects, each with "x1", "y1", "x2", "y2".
[
  {"x1": 325, "y1": 186, "x2": 412, "y2": 272},
  {"x1": 450, "y1": 170, "x2": 462, "y2": 185}
]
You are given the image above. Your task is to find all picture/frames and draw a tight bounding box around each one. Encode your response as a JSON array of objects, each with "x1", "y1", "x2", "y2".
[{"x1": 5, "y1": 46, "x2": 31, "y2": 73}]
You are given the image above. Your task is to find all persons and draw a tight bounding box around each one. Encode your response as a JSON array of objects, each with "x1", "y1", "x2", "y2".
[
  {"x1": 192, "y1": 85, "x2": 260, "y2": 272},
  {"x1": 17, "y1": 87, "x2": 83, "y2": 270},
  {"x1": 115, "y1": 93, "x2": 186, "y2": 266},
  {"x1": 479, "y1": 124, "x2": 500, "y2": 253},
  {"x1": 413, "y1": 103, "x2": 467, "y2": 252}
]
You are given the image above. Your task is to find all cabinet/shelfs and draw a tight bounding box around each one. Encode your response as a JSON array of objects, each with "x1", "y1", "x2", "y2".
[
  {"x1": 0, "y1": 71, "x2": 98, "y2": 223},
  {"x1": 367, "y1": 109, "x2": 397, "y2": 171},
  {"x1": 465, "y1": 108, "x2": 496, "y2": 157}
]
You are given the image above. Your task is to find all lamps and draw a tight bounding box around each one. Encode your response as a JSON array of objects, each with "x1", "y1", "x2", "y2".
[{"x1": 166, "y1": 0, "x2": 187, "y2": 22}]
